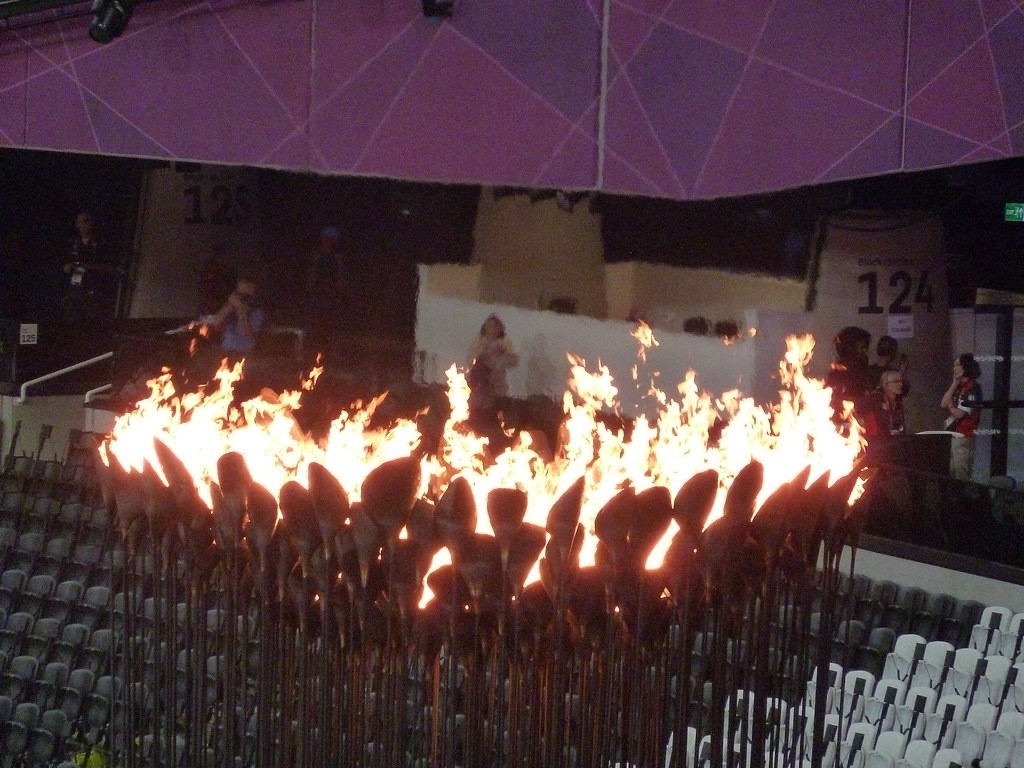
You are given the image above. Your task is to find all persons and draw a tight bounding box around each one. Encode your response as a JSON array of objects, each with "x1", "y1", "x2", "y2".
[
  {"x1": 307, "y1": 225, "x2": 347, "y2": 327},
  {"x1": 872, "y1": 371, "x2": 905, "y2": 460},
  {"x1": 60, "y1": 210, "x2": 113, "y2": 334},
  {"x1": 822, "y1": 326, "x2": 892, "y2": 464},
  {"x1": 203, "y1": 272, "x2": 266, "y2": 356},
  {"x1": 940, "y1": 352, "x2": 983, "y2": 482},
  {"x1": 466, "y1": 316, "x2": 520, "y2": 424},
  {"x1": 867, "y1": 336, "x2": 911, "y2": 402}
]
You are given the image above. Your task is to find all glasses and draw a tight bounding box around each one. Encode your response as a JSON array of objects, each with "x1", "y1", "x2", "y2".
[{"x1": 887, "y1": 379, "x2": 903, "y2": 384}]
